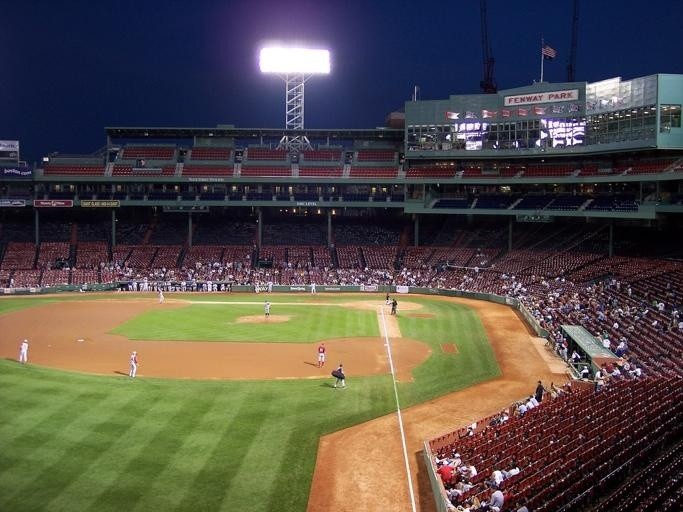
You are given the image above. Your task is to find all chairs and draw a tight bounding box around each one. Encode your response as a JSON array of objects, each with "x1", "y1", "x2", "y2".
[
  {"x1": 439, "y1": 269, "x2": 683, "y2": 309},
  {"x1": 527, "y1": 306, "x2": 683, "y2": 380},
  {"x1": 440, "y1": 380, "x2": 683, "y2": 511}
]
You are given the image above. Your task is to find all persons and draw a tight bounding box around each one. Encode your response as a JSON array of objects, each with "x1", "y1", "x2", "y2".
[
  {"x1": 332, "y1": 363, "x2": 347, "y2": 389},
  {"x1": 18, "y1": 339, "x2": 29, "y2": 363},
  {"x1": 389, "y1": 299, "x2": 397, "y2": 314},
  {"x1": 429, "y1": 361, "x2": 682, "y2": 512},
  {"x1": 128, "y1": 350, "x2": 138, "y2": 378},
  {"x1": 384, "y1": 292, "x2": 390, "y2": 305},
  {"x1": 263, "y1": 300, "x2": 271, "y2": 318},
  {"x1": 316, "y1": 342, "x2": 326, "y2": 368},
  {"x1": 429, "y1": 245, "x2": 564, "y2": 294},
  {"x1": 157, "y1": 288, "x2": 164, "y2": 304},
  {"x1": 522, "y1": 277, "x2": 683, "y2": 367},
  {"x1": 0, "y1": 252, "x2": 429, "y2": 295}
]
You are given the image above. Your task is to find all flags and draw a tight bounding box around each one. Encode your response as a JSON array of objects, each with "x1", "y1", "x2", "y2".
[
  {"x1": 443, "y1": 96, "x2": 624, "y2": 120},
  {"x1": 541, "y1": 44, "x2": 558, "y2": 59}
]
